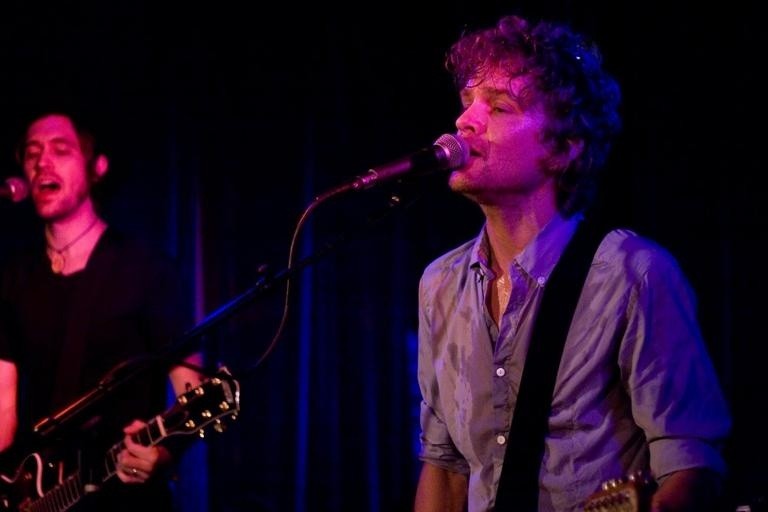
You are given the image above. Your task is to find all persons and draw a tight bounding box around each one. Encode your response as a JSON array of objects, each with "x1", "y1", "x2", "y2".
[
  {"x1": 1, "y1": 103, "x2": 209, "y2": 509},
  {"x1": 405, "y1": 11, "x2": 740, "y2": 512}
]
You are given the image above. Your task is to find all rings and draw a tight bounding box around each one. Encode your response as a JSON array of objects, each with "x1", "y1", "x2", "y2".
[{"x1": 125, "y1": 466, "x2": 140, "y2": 475}]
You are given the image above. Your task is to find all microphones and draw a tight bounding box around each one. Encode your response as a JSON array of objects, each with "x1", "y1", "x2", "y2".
[
  {"x1": 356, "y1": 133, "x2": 471, "y2": 191},
  {"x1": 1, "y1": 177, "x2": 30, "y2": 203}
]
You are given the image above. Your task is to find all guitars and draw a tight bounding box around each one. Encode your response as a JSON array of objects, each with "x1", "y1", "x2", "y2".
[{"x1": 0, "y1": 365, "x2": 241, "y2": 512}]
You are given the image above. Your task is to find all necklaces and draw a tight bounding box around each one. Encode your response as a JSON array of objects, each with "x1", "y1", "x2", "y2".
[{"x1": 31, "y1": 216, "x2": 103, "y2": 274}]
